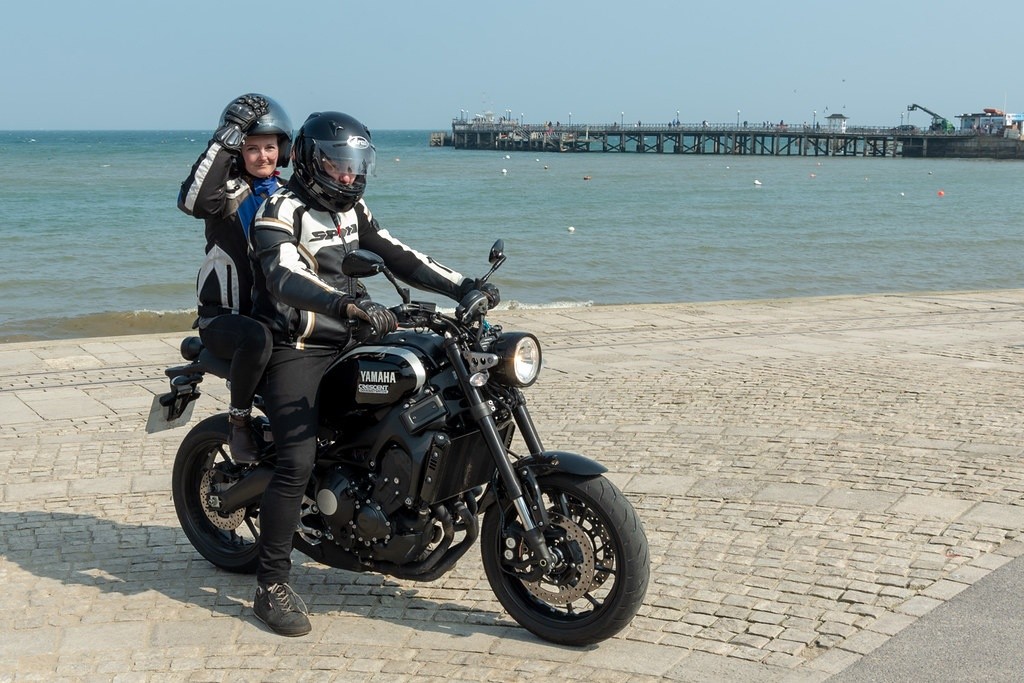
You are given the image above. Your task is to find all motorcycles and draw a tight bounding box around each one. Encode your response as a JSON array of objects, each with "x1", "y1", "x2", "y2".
[{"x1": 157, "y1": 238, "x2": 650, "y2": 648}]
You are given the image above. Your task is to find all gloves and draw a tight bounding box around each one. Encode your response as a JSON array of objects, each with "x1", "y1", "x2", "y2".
[
  {"x1": 212, "y1": 95, "x2": 271, "y2": 158},
  {"x1": 463, "y1": 282, "x2": 501, "y2": 309},
  {"x1": 346, "y1": 297, "x2": 398, "y2": 339}
]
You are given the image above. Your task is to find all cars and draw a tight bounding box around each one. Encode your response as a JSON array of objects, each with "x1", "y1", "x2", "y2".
[{"x1": 891, "y1": 124, "x2": 920, "y2": 135}]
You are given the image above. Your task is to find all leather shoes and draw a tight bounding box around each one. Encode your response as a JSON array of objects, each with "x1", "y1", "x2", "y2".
[{"x1": 227, "y1": 414, "x2": 262, "y2": 463}]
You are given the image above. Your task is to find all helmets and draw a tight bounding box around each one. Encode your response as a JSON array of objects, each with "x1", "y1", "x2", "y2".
[
  {"x1": 219, "y1": 93, "x2": 294, "y2": 174},
  {"x1": 291, "y1": 111, "x2": 376, "y2": 213}
]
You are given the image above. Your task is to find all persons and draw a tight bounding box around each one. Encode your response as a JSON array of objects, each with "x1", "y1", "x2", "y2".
[
  {"x1": 176, "y1": 93, "x2": 295, "y2": 464},
  {"x1": 638, "y1": 119, "x2": 820, "y2": 133},
  {"x1": 557, "y1": 121, "x2": 560, "y2": 127},
  {"x1": 548, "y1": 121, "x2": 552, "y2": 130},
  {"x1": 976, "y1": 123, "x2": 994, "y2": 134},
  {"x1": 251, "y1": 112, "x2": 501, "y2": 636}
]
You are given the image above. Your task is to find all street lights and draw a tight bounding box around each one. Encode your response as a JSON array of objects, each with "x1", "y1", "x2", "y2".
[
  {"x1": 505, "y1": 109, "x2": 511, "y2": 121},
  {"x1": 568, "y1": 112, "x2": 572, "y2": 127},
  {"x1": 737, "y1": 109, "x2": 740, "y2": 128},
  {"x1": 812, "y1": 109, "x2": 817, "y2": 132},
  {"x1": 620, "y1": 111, "x2": 624, "y2": 129},
  {"x1": 521, "y1": 112, "x2": 523, "y2": 126},
  {"x1": 676, "y1": 110, "x2": 679, "y2": 128},
  {"x1": 461, "y1": 109, "x2": 469, "y2": 122}
]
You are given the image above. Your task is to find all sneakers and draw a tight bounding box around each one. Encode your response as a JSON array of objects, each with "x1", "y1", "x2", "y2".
[{"x1": 252, "y1": 584, "x2": 311, "y2": 636}]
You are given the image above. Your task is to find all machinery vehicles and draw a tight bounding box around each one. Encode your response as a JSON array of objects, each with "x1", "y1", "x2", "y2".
[{"x1": 908, "y1": 103, "x2": 956, "y2": 135}]
